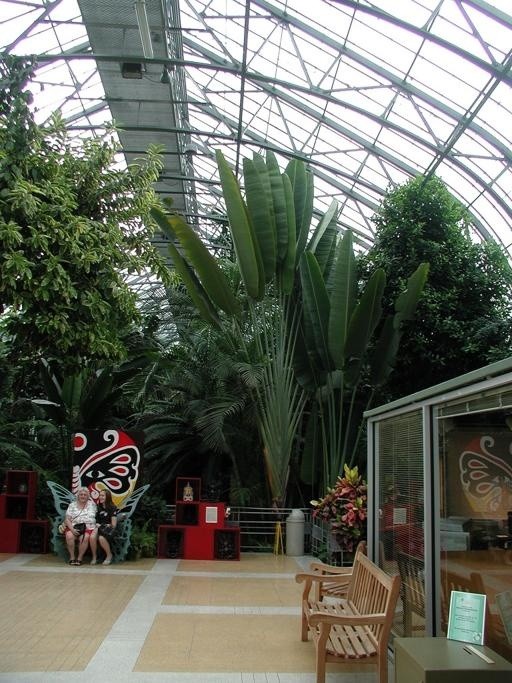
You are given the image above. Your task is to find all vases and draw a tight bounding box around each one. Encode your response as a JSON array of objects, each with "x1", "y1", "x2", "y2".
[{"x1": 328, "y1": 529, "x2": 351, "y2": 550}]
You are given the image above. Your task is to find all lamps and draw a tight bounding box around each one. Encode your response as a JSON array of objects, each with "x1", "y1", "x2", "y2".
[{"x1": 121, "y1": 62, "x2": 142, "y2": 81}]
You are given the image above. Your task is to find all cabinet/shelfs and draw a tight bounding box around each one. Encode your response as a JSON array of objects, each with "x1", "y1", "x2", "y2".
[
  {"x1": 394, "y1": 637, "x2": 512, "y2": 683},
  {"x1": 157, "y1": 477, "x2": 239, "y2": 560},
  {"x1": 0, "y1": 470, "x2": 48, "y2": 554}
]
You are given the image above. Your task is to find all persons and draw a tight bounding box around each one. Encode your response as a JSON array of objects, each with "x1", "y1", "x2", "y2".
[{"x1": 65, "y1": 486, "x2": 118, "y2": 566}]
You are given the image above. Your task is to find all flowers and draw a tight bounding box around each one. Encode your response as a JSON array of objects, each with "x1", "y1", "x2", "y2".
[{"x1": 309, "y1": 462, "x2": 367, "y2": 551}]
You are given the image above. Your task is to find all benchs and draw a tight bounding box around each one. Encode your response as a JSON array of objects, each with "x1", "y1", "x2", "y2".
[
  {"x1": 295, "y1": 541, "x2": 402, "y2": 683},
  {"x1": 397, "y1": 545, "x2": 512, "y2": 660},
  {"x1": 46, "y1": 480, "x2": 150, "y2": 563}
]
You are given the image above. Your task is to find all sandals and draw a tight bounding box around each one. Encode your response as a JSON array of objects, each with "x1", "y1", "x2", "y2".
[
  {"x1": 90, "y1": 561, "x2": 113, "y2": 566},
  {"x1": 69, "y1": 560, "x2": 82, "y2": 566}
]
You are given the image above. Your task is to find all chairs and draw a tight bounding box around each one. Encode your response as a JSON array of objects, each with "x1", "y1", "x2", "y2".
[{"x1": 310, "y1": 541, "x2": 366, "y2": 601}]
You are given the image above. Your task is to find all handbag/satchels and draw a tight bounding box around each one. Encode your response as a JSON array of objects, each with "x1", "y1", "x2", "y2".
[
  {"x1": 58, "y1": 521, "x2": 67, "y2": 534},
  {"x1": 73, "y1": 523, "x2": 86, "y2": 534},
  {"x1": 98, "y1": 522, "x2": 114, "y2": 538}
]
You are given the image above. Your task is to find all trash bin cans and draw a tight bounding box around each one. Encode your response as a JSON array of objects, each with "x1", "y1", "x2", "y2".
[{"x1": 286, "y1": 509, "x2": 305, "y2": 556}]
